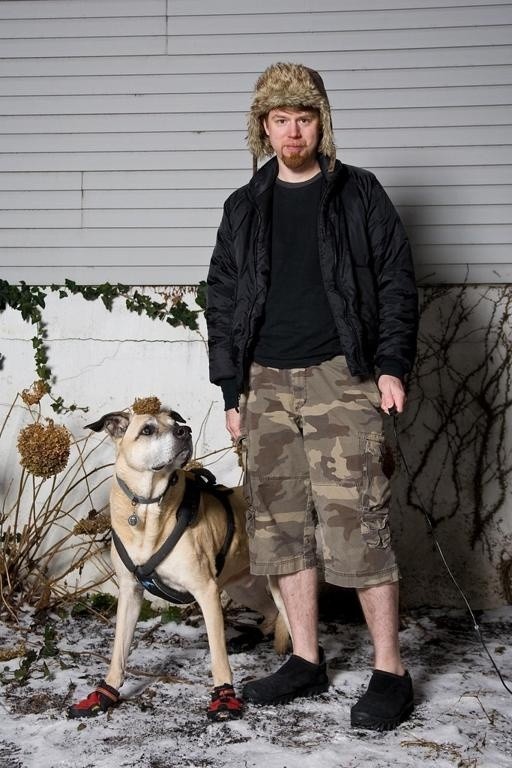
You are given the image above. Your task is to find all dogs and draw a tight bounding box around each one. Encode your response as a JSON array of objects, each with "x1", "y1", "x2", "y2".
[{"x1": 66, "y1": 408, "x2": 290, "y2": 722}]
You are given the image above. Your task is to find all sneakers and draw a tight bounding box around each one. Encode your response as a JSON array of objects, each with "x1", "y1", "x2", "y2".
[
  {"x1": 350, "y1": 668, "x2": 416, "y2": 736},
  {"x1": 242, "y1": 645, "x2": 328, "y2": 704}
]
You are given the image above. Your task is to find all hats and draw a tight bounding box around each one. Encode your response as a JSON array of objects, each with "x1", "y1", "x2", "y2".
[{"x1": 246, "y1": 62, "x2": 336, "y2": 161}]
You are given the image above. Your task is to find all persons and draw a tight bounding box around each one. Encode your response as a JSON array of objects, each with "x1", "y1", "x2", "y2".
[{"x1": 205, "y1": 62, "x2": 419, "y2": 729}]
